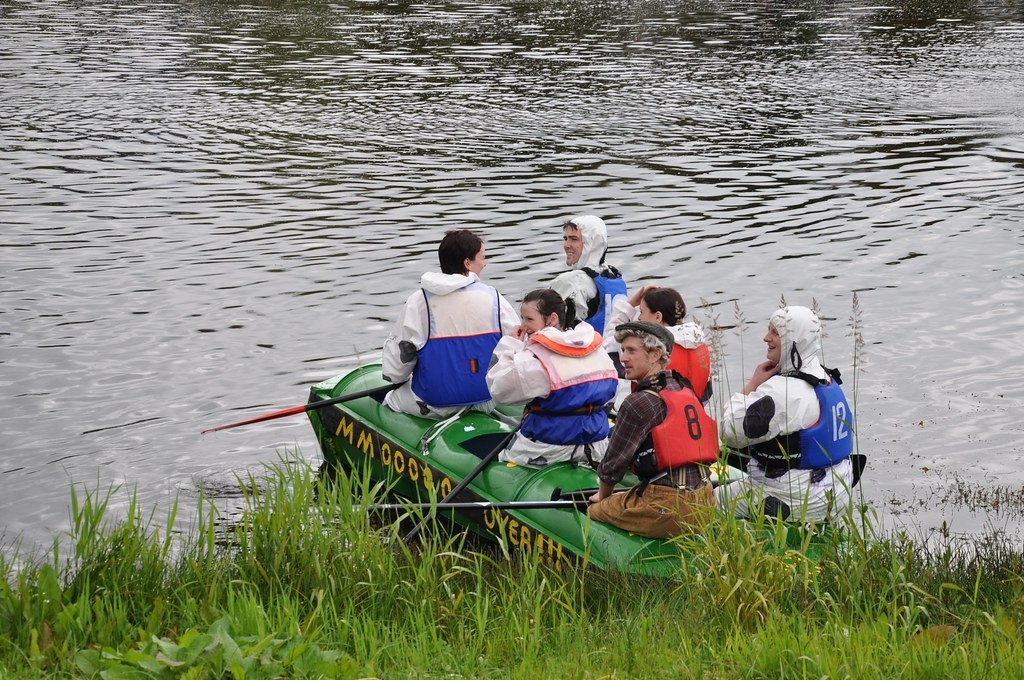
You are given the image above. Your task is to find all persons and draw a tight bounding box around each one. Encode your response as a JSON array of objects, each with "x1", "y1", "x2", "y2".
[
  {"x1": 548, "y1": 215, "x2": 628, "y2": 329},
  {"x1": 713, "y1": 306, "x2": 855, "y2": 525},
  {"x1": 586, "y1": 320, "x2": 717, "y2": 539},
  {"x1": 483, "y1": 288, "x2": 610, "y2": 465},
  {"x1": 602, "y1": 288, "x2": 711, "y2": 416},
  {"x1": 379, "y1": 231, "x2": 523, "y2": 422}
]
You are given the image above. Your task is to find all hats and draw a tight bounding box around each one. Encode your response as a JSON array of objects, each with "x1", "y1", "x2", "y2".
[{"x1": 615, "y1": 320, "x2": 675, "y2": 355}]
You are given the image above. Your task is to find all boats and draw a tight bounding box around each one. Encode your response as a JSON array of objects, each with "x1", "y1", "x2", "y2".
[{"x1": 304, "y1": 359, "x2": 771, "y2": 610}]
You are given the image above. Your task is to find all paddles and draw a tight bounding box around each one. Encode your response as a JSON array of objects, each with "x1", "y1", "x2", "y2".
[
  {"x1": 549, "y1": 478, "x2": 739, "y2": 500},
  {"x1": 384, "y1": 418, "x2": 525, "y2": 572},
  {"x1": 201, "y1": 378, "x2": 405, "y2": 433},
  {"x1": 309, "y1": 497, "x2": 592, "y2": 513}
]
